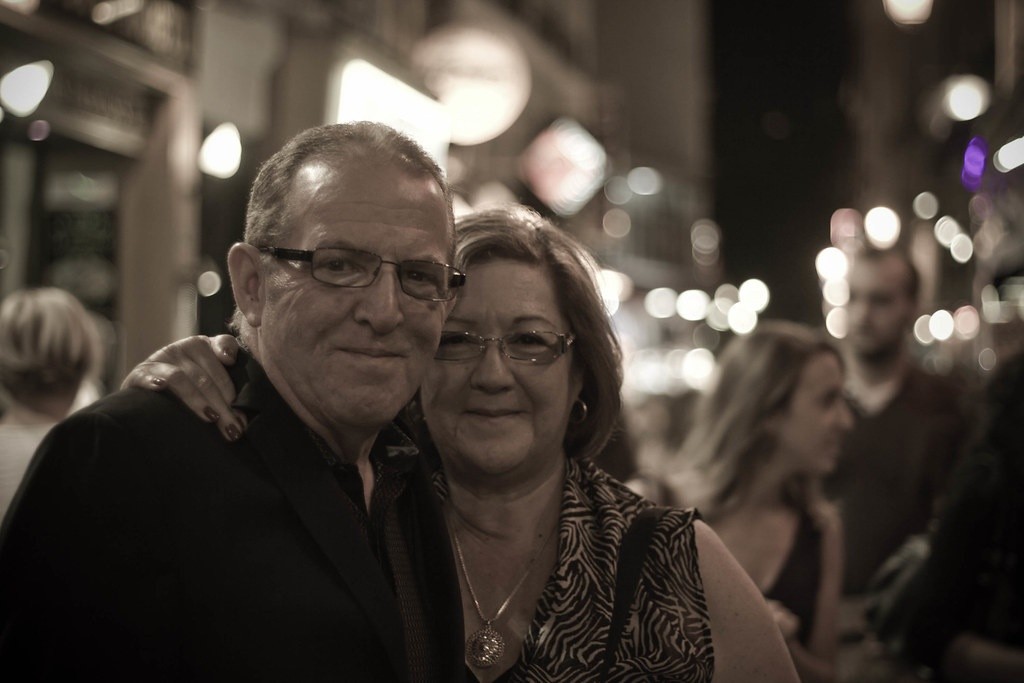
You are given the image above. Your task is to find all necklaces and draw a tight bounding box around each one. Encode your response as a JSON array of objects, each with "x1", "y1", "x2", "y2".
[{"x1": 448, "y1": 514, "x2": 559, "y2": 672}]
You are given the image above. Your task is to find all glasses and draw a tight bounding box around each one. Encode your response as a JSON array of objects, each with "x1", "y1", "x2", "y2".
[
  {"x1": 258, "y1": 246, "x2": 466, "y2": 302},
  {"x1": 433, "y1": 331, "x2": 575, "y2": 365}
]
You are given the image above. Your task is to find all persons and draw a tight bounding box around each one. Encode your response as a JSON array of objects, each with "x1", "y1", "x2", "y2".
[
  {"x1": 116, "y1": 206, "x2": 801, "y2": 682},
  {"x1": 619, "y1": 246, "x2": 1020, "y2": 682},
  {"x1": 1, "y1": 118, "x2": 470, "y2": 682}
]
